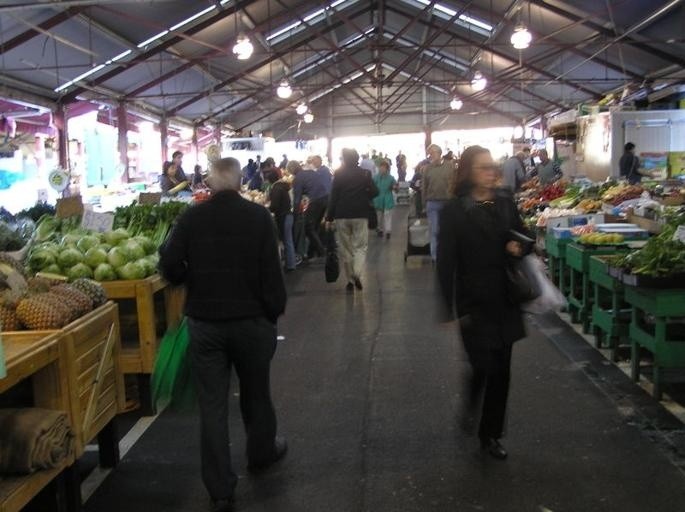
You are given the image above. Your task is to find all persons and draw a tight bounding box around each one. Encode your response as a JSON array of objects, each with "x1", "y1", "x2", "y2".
[
  {"x1": 193, "y1": 163, "x2": 206, "y2": 189},
  {"x1": 359, "y1": 149, "x2": 407, "y2": 182},
  {"x1": 372, "y1": 162, "x2": 399, "y2": 240},
  {"x1": 240, "y1": 154, "x2": 332, "y2": 273},
  {"x1": 160, "y1": 151, "x2": 192, "y2": 198},
  {"x1": 159, "y1": 157, "x2": 288, "y2": 511},
  {"x1": 411, "y1": 145, "x2": 459, "y2": 268},
  {"x1": 496, "y1": 146, "x2": 563, "y2": 203},
  {"x1": 617, "y1": 139, "x2": 656, "y2": 187},
  {"x1": 325, "y1": 148, "x2": 380, "y2": 290},
  {"x1": 429, "y1": 144, "x2": 542, "y2": 461}
]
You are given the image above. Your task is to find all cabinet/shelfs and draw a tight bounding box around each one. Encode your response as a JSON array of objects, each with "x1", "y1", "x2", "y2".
[
  {"x1": 0, "y1": 297, "x2": 128, "y2": 510},
  {"x1": 549, "y1": 216, "x2": 685, "y2": 402},
  {"x1": 94, "y1": 269, "x2": 186, "y2": 418}
]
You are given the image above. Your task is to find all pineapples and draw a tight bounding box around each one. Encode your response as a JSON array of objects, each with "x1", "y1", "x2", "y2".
[{"x1": 0, "y1": 251, "x2": 106, "y2": 330}]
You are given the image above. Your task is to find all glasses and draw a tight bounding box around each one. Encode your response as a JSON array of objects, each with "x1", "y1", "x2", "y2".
[{"x1": 472, "y1": 164, "x2": 498, "y2": 172}]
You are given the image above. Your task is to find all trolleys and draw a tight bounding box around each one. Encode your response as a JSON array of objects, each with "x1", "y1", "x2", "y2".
[{"x1": 403, "y1": 191, "x2": 432, "y2": 263}]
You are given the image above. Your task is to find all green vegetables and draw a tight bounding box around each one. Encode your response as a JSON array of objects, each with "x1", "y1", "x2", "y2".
[{"x1": 126, "y1": 200, "x2": 188, "y2": 248}]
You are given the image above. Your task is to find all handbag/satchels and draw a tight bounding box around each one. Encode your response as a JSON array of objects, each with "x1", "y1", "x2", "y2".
[
  {"x1": 56, "y1": 189, "x2": 82, "y2": 218},
  {"x1": 368, "y1": 204, "x2": 379, "y2": 230},
  {"x1": 508, "y1": 260, "x2": 543, "y2": 305},
  {"x1": 324, "y1": 250, "x2": 340, "y2": 284}
]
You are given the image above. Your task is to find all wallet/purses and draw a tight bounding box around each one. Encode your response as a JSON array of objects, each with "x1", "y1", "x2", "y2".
[{"x1": 508, "y1": 230, "x2": 535, "y2": 255}]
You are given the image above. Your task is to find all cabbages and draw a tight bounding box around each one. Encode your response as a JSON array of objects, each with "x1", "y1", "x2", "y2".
[{"x1": 23, "y1": 228, "x2": 160, "y2": 282}]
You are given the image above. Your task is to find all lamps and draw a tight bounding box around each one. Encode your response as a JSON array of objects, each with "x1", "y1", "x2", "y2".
[
  {"x1": 275, "y1": 58, "x2": 293, "y2": 101},
  {"x1": 228, "y1": 2, "x2": 255, "y2": 61},
  {"x1": 295, "y1": 87, "x2": 315, "y2": 125},
  {"x1": 509, "y1": 0, "x2": 533, "y2": 51},
  {"x1": 471, "y1": 49, "x2": 487, "y2": 92},
  {"x1": 449, "y1": 22, "x2": 463, "y2": 112}
]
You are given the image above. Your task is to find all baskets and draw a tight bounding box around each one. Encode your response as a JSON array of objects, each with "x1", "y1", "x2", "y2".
[
  {"x1": 654, "y1": 197, "x2": 683, "y2": 206},
  {"x1": 138, "y1": 192, "x2": 160, "y2": 205}
]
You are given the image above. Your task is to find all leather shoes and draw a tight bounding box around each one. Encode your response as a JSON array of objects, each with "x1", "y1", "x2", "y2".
[
  {"x1": 351, "y1": 274, "x2": 363, "y2": 291},
  {"x1": 208, "y1": 497, "x2": 235, "y2": 512},
  {"x1": 272, "y1": 435, "x2": 288, "y2": 463},
  {"x1": 478, "y1": 434, "x2": 507, "y2": 459}
]
